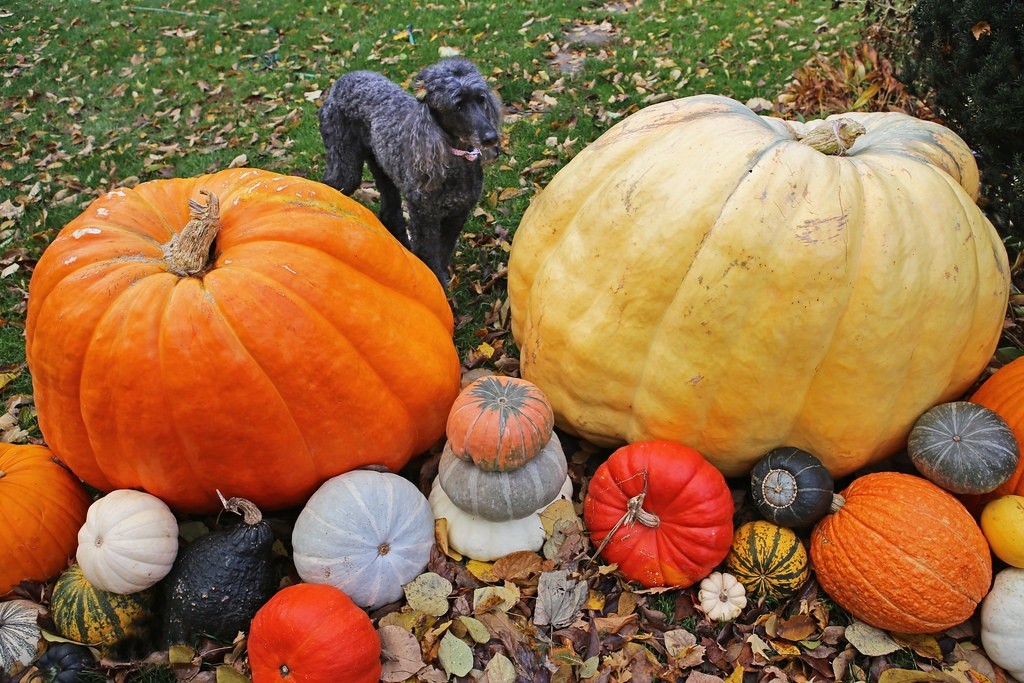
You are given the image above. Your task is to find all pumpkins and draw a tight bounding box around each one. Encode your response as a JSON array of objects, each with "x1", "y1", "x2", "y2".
[{"x1": 0, "y1": 93, "x2": 1023, "y2": 683}]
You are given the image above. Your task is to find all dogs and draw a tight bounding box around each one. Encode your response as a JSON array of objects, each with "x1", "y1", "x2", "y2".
[{"x1": 317, "y1": 59, "x2": 504, "y2": 291}]
